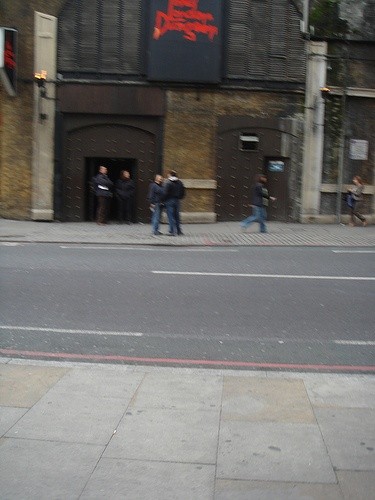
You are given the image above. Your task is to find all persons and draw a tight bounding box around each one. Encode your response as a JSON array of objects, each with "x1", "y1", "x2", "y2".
[
  {"x1": 163, "y1": 169, "x2": 185, "y2": 236},
  {"x1": 239, "y1": 174, "x2": 277, "y2": 234},
  {"x1": 96, "y1": 166, "x2": 114, "y2": 226},
  {"x1": 348, "y1": 175, "x2": 368, "y2": 228},
  {"x1": 148, "y1": 174, "x2": 167, "y2": 237},
  {"x1": 116, "y1": 170, "x2": 140, "y2": 225}
]
